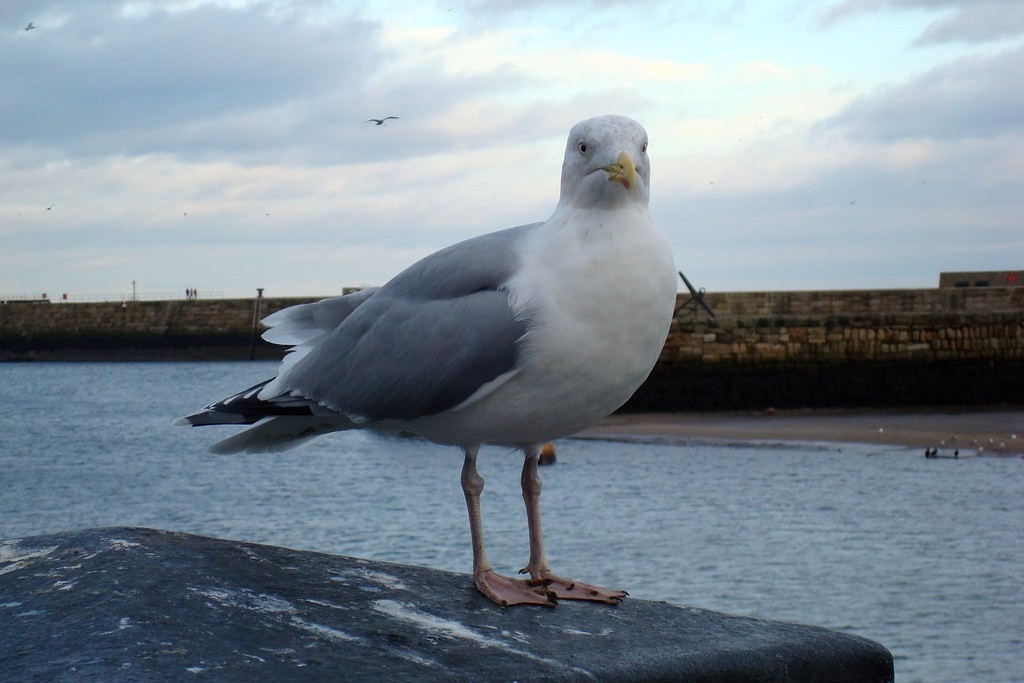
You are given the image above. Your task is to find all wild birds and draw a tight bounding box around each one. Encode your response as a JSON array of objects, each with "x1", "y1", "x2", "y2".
[
  {"x1": 25, "y1": 21, "x2": 37, "y2": 33},
  {"x1": 171, "y1": 115, "x2": 679, "y2": 609},
  {"x1": 44, "y1": 203, "x2": 54, "y2": 212},
  {"x1": 876, "y1": 422, "x2": 1018, "y2": 459}
]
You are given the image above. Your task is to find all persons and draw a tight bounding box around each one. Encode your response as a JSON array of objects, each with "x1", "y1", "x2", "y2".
[
  {"x1": 189, "y1": 287, "x2": 197, "y2": 300},
  {"x1": 185, "y1": 288, "x2": 190, "y2": 300}
]
